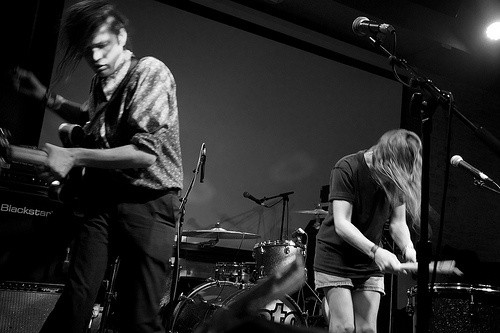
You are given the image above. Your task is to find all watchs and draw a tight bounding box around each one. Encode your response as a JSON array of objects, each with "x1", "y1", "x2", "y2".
[{"x1": 367, "y1": 245, "x2": 379, "y2": 260}]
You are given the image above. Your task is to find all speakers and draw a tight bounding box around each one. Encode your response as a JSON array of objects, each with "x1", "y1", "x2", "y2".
[{"x1": 0, "y1": 280, "x2": 109, "y2": 333}]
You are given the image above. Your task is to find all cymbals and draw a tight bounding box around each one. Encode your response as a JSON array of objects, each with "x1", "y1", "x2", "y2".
[
  {"x1": 175, "y1": 227, "x2": 263, "y2": 239},
  {"x1": 291, "y1": 209, "x2": 328, "y2": 215}
]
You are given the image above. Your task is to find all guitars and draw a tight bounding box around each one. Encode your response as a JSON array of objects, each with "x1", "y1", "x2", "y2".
[{"x1": 0, "y1": 123, "x2": 103, "y2": 204}]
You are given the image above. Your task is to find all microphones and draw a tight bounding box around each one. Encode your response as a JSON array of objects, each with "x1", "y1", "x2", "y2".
[
  {"x1": 243, "y1": 192, "x2": 265, "y2": 206},
  {"x1": 200, "y1": 144, "x2": 207, "y2": 183},
  {"x1": 352, "y1": 16, "x2": 396, "y2": 36},
  {"x1": 450, "y1": 154, "x2": 494, "y2": 183}
]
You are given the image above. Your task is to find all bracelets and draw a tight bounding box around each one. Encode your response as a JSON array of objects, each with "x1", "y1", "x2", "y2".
[
  {"x1": 401, "y1": 245, "x2": 414, "y2": 260},
  {"x1": 40, "y1": 87, "x2": 51, "y2": 103},
  {"x1": 52, "y1": 94, "x2": 63, "y2": 111}
]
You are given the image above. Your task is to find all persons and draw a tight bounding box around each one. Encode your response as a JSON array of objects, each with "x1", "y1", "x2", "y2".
[
  {"x1": 314, "y1": 129, "x2": 426, "y2": 333},
  {"x1": 14, "y1": 0, "x2": 183, "y2": 332}
]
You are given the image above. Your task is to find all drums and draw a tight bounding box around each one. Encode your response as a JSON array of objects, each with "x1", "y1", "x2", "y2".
[
  {"x1": 169, "y1": 280, "x2": 308, "y2": 333},
  {"x1": 215, "y1": 261, "x2": 257, "y2": 284},
  {"x1": 252, "y1": 240, "x2": 307, "y2": 278},
  {"x1": 411, "y1": 283, "x2": 500, "y2": 333}
]
